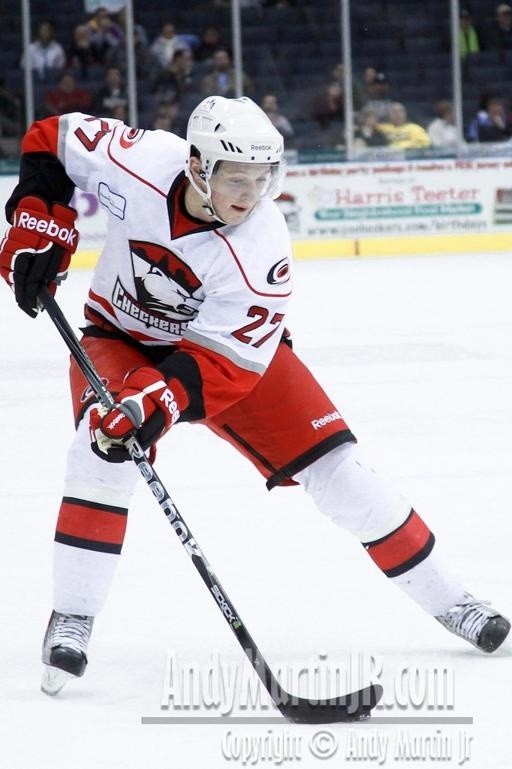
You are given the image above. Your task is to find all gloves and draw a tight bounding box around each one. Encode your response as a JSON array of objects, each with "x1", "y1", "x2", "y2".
[
  {"x1": 89, "y1": 365, "x2": 188, "y2": 464},
  {"x1": 1, "y1": 195, "x2": 78, "y2": 318}
]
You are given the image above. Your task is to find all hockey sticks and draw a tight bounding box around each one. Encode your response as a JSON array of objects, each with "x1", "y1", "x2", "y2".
[{"x1": 39, "y1": 285, "x2": 383, "y2": 724}]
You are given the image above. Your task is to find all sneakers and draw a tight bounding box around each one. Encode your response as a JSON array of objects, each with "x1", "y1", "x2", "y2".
[
  {"x1": 41, "y1": 609, "x2": 104, "y2": 677},
  {"x1": 434, "y1": 592, "x2": 509, "y2": 655}
]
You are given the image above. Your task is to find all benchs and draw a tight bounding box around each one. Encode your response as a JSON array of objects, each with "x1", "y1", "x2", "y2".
[{"x1": 1, "y1": 2, "x2": 512, "y2": 147}]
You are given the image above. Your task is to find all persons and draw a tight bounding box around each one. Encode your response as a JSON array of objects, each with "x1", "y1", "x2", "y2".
[
  {"x1": 0, "y1": 95, "x2": 509, "y2": 696},
  {"x1": 0, "y1": 1, "x2": 510, "y2": 151}
]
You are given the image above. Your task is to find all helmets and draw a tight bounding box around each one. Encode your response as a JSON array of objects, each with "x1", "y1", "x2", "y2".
[{"x1": 185, "y1": 94, "x2": 287, "y2": 202}]
